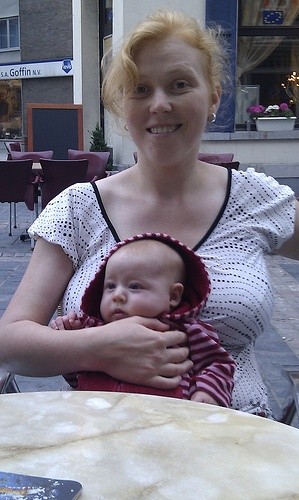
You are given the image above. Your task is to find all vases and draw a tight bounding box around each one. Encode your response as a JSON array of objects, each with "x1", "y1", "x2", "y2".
[{"x1": 252, "y1": 116, "x2": 297, "y2": 132}]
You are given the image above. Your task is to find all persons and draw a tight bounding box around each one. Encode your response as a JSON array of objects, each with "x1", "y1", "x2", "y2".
[
  {"x1": 49, "y1": 233, "x2": 234, "y2": 410},
  {"x1": 0, "y1": 10, "x2": 296, "y2": 427}
]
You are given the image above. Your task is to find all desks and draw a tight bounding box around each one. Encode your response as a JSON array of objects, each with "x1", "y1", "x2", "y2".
[
  {"x1": 0, "y1": 390, "x2": 299, "y2": 500},
  {"x1": 14, "y1": 163, "x2": 44, "y2": 251}
]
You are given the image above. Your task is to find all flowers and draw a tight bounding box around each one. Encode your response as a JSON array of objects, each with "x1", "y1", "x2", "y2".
[{"x1": 246, "y1": 102, "x2": 295, "y2": 119}]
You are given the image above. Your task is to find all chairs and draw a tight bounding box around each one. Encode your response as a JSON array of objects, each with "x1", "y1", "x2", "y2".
[
  {"x1": 39, "y1": 158, "x2": 89, "y2": 213},
  {"x1": 0, "y1": 159, "x2": 41, "y2": 237},
  {"x1": 9, "y1": 142, "x2": 22, "y2": 152},
  {"x1": 67, "y1": 149, "x2": 111, "y2": 182},
  {"x1": 197, "y1": 152, "x2": 240, "y2": 171},
  {"x1": 7, "y1": 151, "x2": 54, "y2": 166}
]
